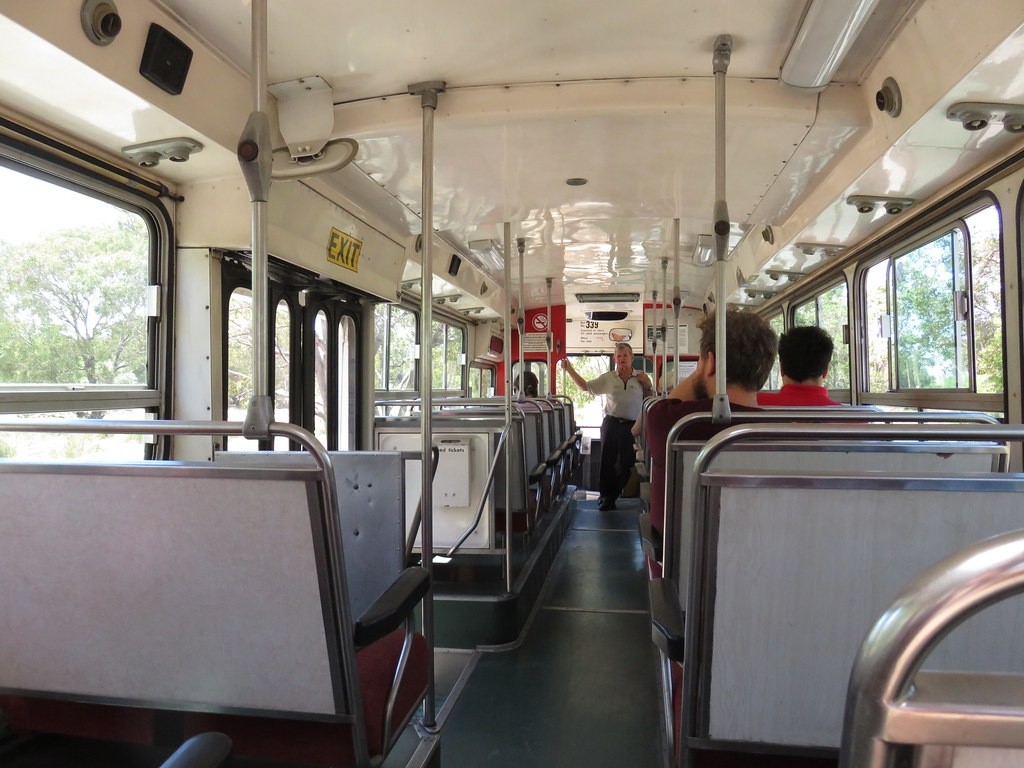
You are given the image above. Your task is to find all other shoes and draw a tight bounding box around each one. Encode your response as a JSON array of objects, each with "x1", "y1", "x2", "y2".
[{"x1": 597, "y1": 498, "x2": 615, "y2": 511}]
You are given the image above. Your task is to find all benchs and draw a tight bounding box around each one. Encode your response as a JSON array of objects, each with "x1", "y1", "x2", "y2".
[{"x1": 0, "y1": 394, "x2": 1024, "y2": 767}]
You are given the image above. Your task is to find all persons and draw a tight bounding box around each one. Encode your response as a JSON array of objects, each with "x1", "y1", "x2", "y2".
[
  {"x1": 562, "y1": 343, "x2": 652, "y2": 511},
  {"x1": 756, "y1": 326, "x2": 869, "y2": 424},
  {"x1": 513, "y1": 371, "x2": 538, "y2": 408},
  {"x1": 645, "y1": 309, "x2": 778, "y2": 579}
]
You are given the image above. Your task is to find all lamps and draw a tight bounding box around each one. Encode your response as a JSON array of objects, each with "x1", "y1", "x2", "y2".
[
  {"x1": 778, "y1": 0, "x2": 880, "y2": 93},
  {"x1": 691, "y1": 234, "x2": 715, "y2": 267},
  {"x1": 467, "y1": 239, "x2": 505, "y2": 272}
]
